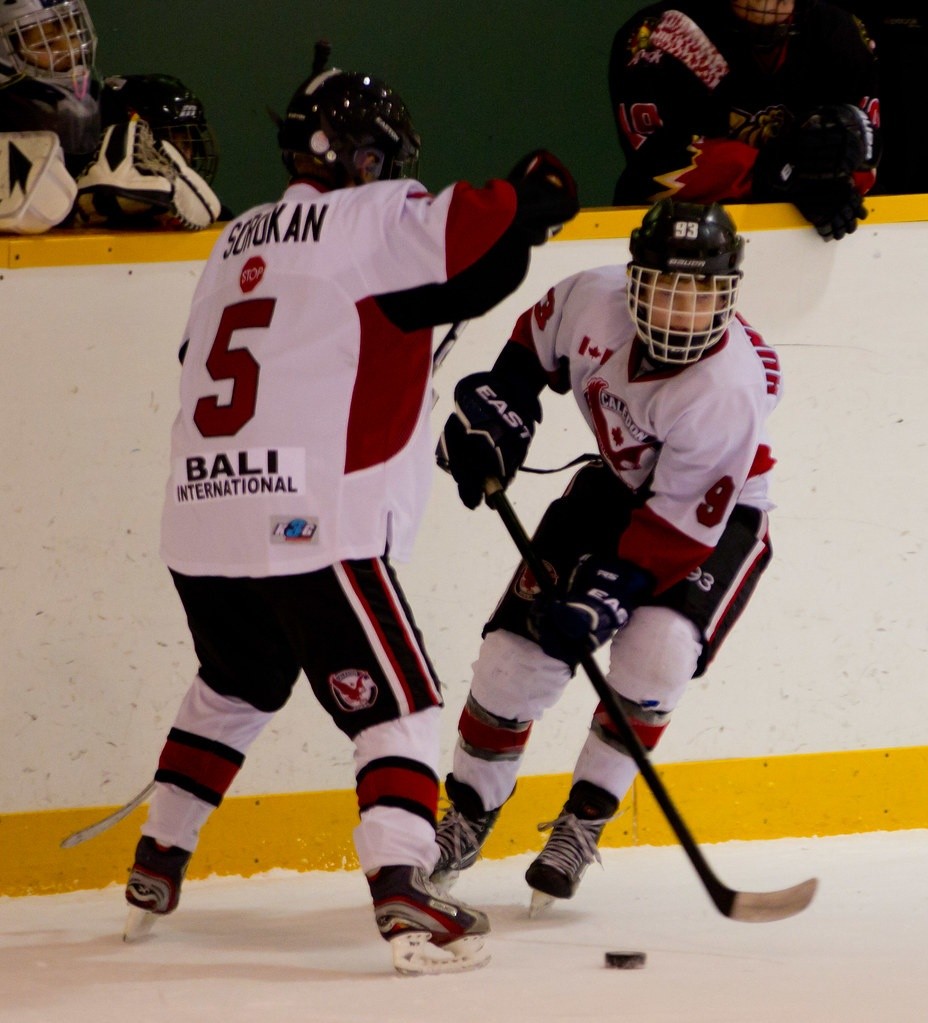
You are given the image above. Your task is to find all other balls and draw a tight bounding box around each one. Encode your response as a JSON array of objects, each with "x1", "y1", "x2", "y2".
[{"x1": 606, "y1": 948, "x2": 646, "y2": 969}]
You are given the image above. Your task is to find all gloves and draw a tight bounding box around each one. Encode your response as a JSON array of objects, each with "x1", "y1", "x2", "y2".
[
  {"x1": 751, "y1": 152, "x2": 868, "y2": 243},
  {"x1": 434, "y1": 370, "x2": 543, "y2": 510},
  {"x1": 805, "y1": 99, "x2": 882, "y2": 173},
  {"x1": 507, "y1": 147, "x2": 580, "y2": 230},
  {"x1": 526, "y1": 551, "x2": 660, "y2": 664}
]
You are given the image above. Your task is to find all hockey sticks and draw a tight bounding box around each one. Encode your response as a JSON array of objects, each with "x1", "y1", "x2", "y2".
[
  {"x1": 427, "y1": 315, "x2": 817, "y2": 923},
  {"x1": 53, "y1": 319, "x2": 467, "y2": 855}
]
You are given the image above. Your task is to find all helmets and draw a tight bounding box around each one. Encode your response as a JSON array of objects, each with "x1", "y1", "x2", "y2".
[
  {"x1": 0, "y1": 0, "x2": 97, "y2": 81},
  {"x1": 624, "y1": 195, "x2": 744, "y2": 284},
  {"x1": 278, "y1": 68, "x2": 419, "y2": 180}
]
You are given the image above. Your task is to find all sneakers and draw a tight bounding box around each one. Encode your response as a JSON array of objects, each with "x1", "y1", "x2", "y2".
[
  {"x1": 524, "y1": 780, "x2": 620, "y2": 917},
  {"x1": 121, "y1": 835, "x2": 193, "y2": 943},
  {"x1": 427, "y1": 772, "x2": 524, "y2": 885},
  {"x1": 362, "y1": 866, "x2": 491, "y2": 978}
]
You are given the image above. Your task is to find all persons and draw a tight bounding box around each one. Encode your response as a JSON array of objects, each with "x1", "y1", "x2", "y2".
[
  {"x1": 610, "y1": 0, "x2": 892, "y2": 245},
  {"x1": 121, "y1": 67, "x2": 583, "y2": 976},
  {"x1": 0, "y1": 1, "x2": 236, "y2": 235},
  {"x1": 435, "y1": 193, "x2": 782, "y2": 913}
]
